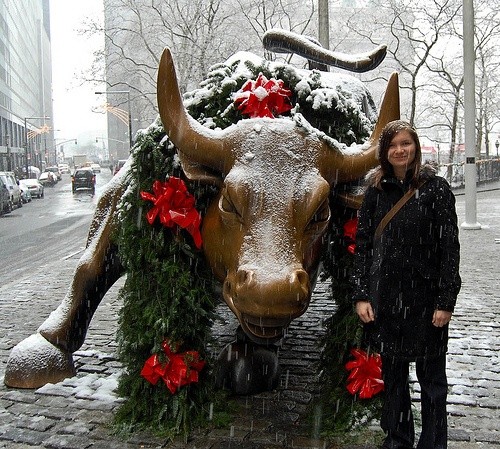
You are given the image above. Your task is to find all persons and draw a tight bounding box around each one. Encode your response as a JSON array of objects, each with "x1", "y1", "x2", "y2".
[{"x1": 353, "y1": 121, "x2": 462, "y2": 449}]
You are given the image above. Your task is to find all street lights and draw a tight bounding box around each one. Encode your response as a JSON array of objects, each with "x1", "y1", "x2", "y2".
[
  {"x1": 96, "y1": 137, "x2": 126, "y2": 152},
  {"x1": 25, "y1": 117, "x2": 51, "y2": 172},
  {"x1": 94, "y1": 91, "x2": 133, "y2": 154},
  {"x1": 55, "y1": 139, "x2": 77, "y2": 163}
]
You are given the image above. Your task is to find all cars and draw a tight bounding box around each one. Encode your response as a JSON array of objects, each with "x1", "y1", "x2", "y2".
[
  {"x1": 58, "y1": 154, "x2": 127, "y2": 175},
  {"x1": 44, "y1": 167, "x2": 61, "y2": 179},
  {"x1": 70, "y1": 170, "x2": 96, "y2": 191},
  {"x1": 39, "y1": 172, "x2": 58, "y2": 185},
  {"x1": 0, "y1": 172, "x2": 32, "y2": 214},
  {"x1": 19, "y1": 179, "x2": 43, "y2": 198}
]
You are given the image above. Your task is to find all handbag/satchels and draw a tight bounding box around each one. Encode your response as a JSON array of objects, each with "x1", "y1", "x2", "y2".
[{"x1": 368, "y1": 259, "x2": 391, "y2": 309}]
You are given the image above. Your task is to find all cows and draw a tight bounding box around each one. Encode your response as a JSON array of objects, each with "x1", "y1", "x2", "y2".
[{"x1": 5, "y1": 28, "x2": 401, "y2": 391}]
